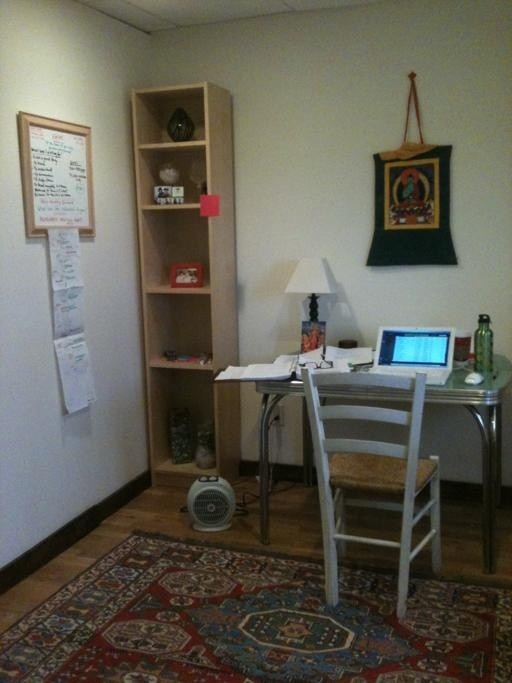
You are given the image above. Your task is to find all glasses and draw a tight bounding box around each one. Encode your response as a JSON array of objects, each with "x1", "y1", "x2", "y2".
[{"x1": 298, "y1": 360, "x2": 333, "y2": 368}]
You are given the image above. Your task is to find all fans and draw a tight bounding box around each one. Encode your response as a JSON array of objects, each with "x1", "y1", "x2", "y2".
[{"x1": 187, "y1": 475, "x2": 236, "y2": 532}]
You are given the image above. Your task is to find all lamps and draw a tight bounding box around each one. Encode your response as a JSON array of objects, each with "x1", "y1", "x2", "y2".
[{"x1": 285, "y1": 257, "x2": 340, "y2": 351}]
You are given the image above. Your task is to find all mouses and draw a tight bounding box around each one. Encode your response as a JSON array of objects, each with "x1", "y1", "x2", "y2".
[{"x1": 465, "y1": 370, "x2": 483, "y2": 387}]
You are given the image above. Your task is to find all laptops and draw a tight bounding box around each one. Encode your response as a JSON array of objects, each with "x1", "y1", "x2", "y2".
[{"x1": 369, "y1": 325, "x2": 454, "y2": 386}]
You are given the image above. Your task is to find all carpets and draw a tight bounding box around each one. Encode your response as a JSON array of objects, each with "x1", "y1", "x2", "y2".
[{"x1": 0, "y1": 528, "x2": 512, "y2": 683}]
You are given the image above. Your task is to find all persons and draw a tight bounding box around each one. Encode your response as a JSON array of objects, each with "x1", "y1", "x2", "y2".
[
  {"x1": 157, "y1": 187, "x2": 171, "y2": 198},
  {"x1": 174, "y1": 269, "x2": 199, "y2": 284}
]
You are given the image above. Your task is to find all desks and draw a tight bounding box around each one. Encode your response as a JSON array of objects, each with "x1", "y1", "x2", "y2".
[{"x1": 255, "y1": 354, "x2": 512, "y2": 573}]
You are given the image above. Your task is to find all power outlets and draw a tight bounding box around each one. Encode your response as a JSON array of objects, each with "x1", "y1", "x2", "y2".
[{"x1": 271, "y1": 405, "x2": 284, "y2": 427}]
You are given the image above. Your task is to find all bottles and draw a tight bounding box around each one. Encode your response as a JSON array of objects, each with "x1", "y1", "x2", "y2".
[
  {"x1": 454, "y1": 330, "x2": 472, "y2": 367},
  {"x1": 474, "y1": 314, "x2": 493, "y2": 374}
]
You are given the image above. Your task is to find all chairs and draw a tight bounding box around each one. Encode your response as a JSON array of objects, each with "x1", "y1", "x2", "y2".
[{"x1": 303, "y1": 370, "x2": 451, "y2": 621}]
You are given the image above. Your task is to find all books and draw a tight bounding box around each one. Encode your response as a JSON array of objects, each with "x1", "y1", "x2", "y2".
[{"x1": 214, "y1": 346, "x2": 373, "y2": 382}]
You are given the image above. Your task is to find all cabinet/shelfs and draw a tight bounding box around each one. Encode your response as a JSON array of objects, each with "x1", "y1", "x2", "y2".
[{"x1": 130, "y1": 80, "x2": 242, "y2": 487}]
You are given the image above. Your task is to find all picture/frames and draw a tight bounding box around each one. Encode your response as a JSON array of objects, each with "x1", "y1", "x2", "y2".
[{"x1": 171, "y1": 263, "x2": 203, "y2": 288}]
